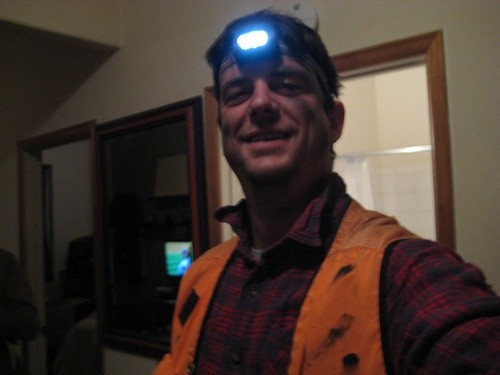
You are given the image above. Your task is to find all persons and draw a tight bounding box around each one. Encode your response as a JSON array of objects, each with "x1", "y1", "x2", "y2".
[
  {"x1": 150, "y1": 13, "x2": 500, "y2": 375},
  {"x1": 0, "y1": 248, "x2": 42, "y2": 375}
]
